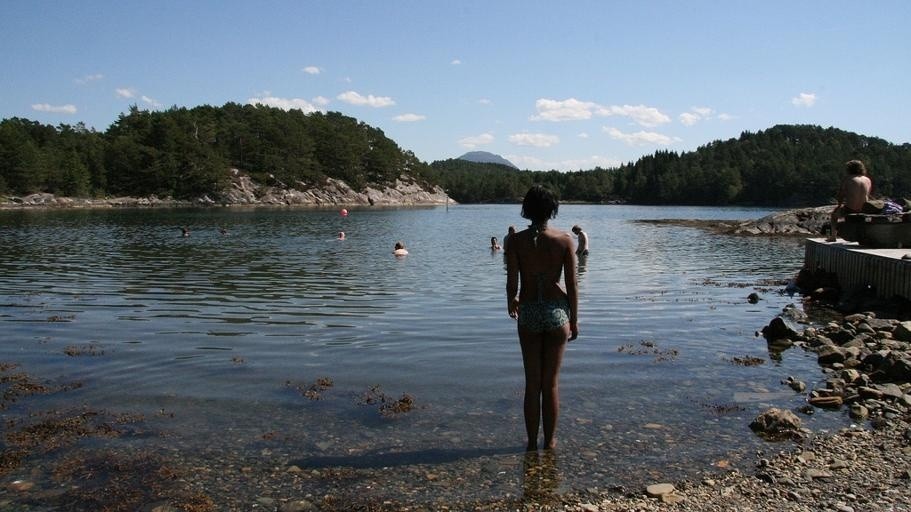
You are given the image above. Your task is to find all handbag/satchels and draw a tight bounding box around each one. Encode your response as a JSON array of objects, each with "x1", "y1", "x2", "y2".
[{"x1": 863, "y1": 199, "x2": 884, "y2": 214}]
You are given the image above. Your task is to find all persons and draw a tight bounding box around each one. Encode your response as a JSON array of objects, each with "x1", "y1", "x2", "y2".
[
  {"x1": 570, "y1": 225, "x2": 588, "y2": 256},
  {"x1": 824, "y1": 158, "x2": 872, "y2": 242},
  {"x1": 504, "y1": 184, "x2": 578, "y2": 452},
  {"x1": 502, "y1": 225, "x2": 514, "y2": 265},
  {"x1": 390, "y1": 241, "x2": 410, "y2": 258},
  {"x1": 576, "y1": 257, "x2": 587, "y2": 287},
  {"x1": 489, "y1": 236, "x2": 501, "y2": 250},
  {"x1": 180, "y1": 228, "x2": 190, "y2": 237},
  {"x1": 338, "y1": 230, "x2": 348, "y2": 241}
]
